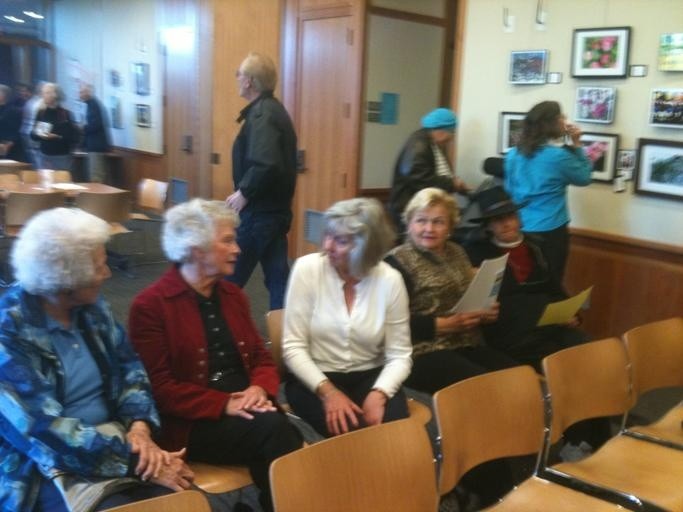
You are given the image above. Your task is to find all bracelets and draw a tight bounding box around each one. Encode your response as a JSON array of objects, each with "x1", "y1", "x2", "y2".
[
  {"x1": 316, "y1": 379, "x2": 332, "y2": 395},
  {"x1": 368, "y1": 388, "x2": 389, "y2": 404}
]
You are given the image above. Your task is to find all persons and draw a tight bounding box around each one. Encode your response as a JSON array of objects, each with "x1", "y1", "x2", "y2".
[
  {"x1": 467, "y1": 185, "x2": 611, "y2": 463},
  {"x1": 386, "y1": 108, "x2": 474, "y2": 246},
  {"x1": 502, "y1": 101, "x2": 592, "y2": 283},
  {"x1": 222, "y1": 51, "x2": 297, "y2": 311},
  {"x1": 0, "y1": 81, "x2": 114, "y2": 183},
  {"x1": 383, "y1": 187, "x2": 519, "y2": 506},
  {"x1": 129, "y1": 197, "x2": 304, "y2": 512},
  {"x1": 280, "y1": 197, "x2": 414, "y2": 438},
  {"x1": 0, "y1": 205, "x2": 195, "y2": 512}
]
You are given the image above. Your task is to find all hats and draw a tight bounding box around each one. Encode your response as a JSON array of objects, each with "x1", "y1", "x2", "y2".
[
  {"x1": 468, "y1": 187, "x2": 528, "y2": 222},
  {"x1": 423, "y1": 108, "x2": 456, "y2": 128}
]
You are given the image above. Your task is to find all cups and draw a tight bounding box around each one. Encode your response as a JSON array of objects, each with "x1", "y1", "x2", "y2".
[{"x1": 40, "y1": 168, "x2": 54, "y2": 186}]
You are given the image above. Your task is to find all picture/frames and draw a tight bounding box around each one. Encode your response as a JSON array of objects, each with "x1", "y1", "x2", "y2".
[
  {"x1": 567, "y1": 21, "x2": 631, "y2": 81},
  {"x1": 496, "y1": 107, "x2": 535, "y2": 155},
  {"x1": 646, "y1": 84, "x2": 682, "y2": 128},
  {"x1": 633, "y1": 137, "x2": 683, "y2": 202},
  {"x1": 104, "y1": 61, "x2": 152, "y2": 132},
  {"x1": 507, "y1": 47, "x2": 550, "y2": 87},
  {"x1": 571, "y1": 83, "x2": 614, "y2": 126},
  {"x1": 563, "y1": 128, "x2": 618, "y2": 185}
]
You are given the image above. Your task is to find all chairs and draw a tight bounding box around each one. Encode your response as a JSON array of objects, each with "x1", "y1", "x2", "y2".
[
  {"x1": 623, "y1": 310, "x2": 682, "y2": 448},
  {"x1": 97, "y1": 487, "x2": 214, "y2": 512},
  {"x1": 267, "y1": 413, "x2": 444, "y2": 512},
  {"x1": 422, "y1": 362, "x2": 640, "y2": 512},
  {"x1": 262, "y1": 304, "x2": 435, "y2": 435},
  {"x1": 0, "y1": 157, "x2": 173, "y2": 283},
  {"x1": 536, "y1": 335, "x2": 683, "y2": 512},
  {"x1": 179, "y1": 439, "x2": 310, "y2": 510}
]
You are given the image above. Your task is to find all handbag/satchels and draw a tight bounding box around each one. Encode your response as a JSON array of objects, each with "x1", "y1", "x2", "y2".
[{"x1": 37, "y1": 422, "x2": 146, "y2": 511}]
